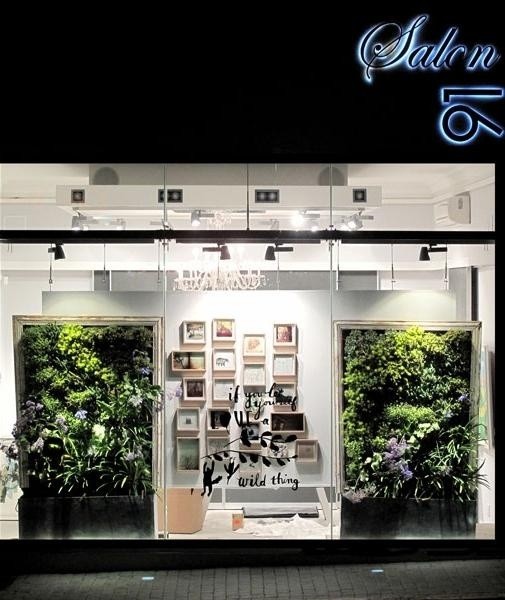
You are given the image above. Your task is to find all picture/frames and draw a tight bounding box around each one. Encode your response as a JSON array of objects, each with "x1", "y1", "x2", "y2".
[{"x1": 170, "y1": 317, "x2": 319, "y2": 475}]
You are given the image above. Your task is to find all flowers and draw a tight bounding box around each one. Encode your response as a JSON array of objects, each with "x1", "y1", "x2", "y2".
[
  {"x1": 0, "y1": 324, "x2": 163, "y2": 508},
  {"x1": 337, "y1": 322, "x2": 493, "y2": 506}
]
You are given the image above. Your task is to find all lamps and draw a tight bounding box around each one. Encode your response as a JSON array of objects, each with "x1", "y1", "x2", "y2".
[
  {"x1": 48, "y1": 244, "x2": 65, "y2": 260},
  {"x1": 190, "y1": 211, "x2": 214, "y2": 226},
  {"x1": 71, "y1": 215, "x2": 88, "y2": 231},
  {"x1": 419, "y1": 244, "x2": 448, "y2": 262},
  {"x1": 265, "y1": 242, "x2": 294, "y2": 260},
  {"x1": 203, "y1": 241, "x2": 231, "y2": 261}
]
